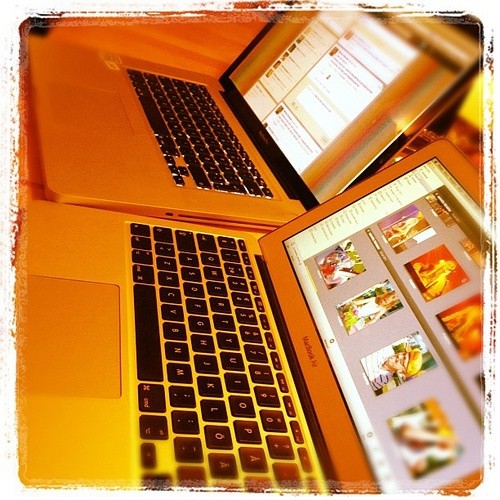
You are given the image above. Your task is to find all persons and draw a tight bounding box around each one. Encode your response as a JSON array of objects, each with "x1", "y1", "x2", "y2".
[
  {"x1": 325, "y1": 241, "x2": 367, "y2": 287},
  {"x1": 360, "y1": 345, "x2": 422, "y2": 391},
  {"x1": 349, "y1": 290, "x2": 401, "y2": 326}
]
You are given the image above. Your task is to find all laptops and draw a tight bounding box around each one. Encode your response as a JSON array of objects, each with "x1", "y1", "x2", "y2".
[
  {"x1": 26, "y1": 5, "x2": 494, "y2": 231},
  {"x1": 14, "y1": 139, "x2": 496, "y2": 495}
]
